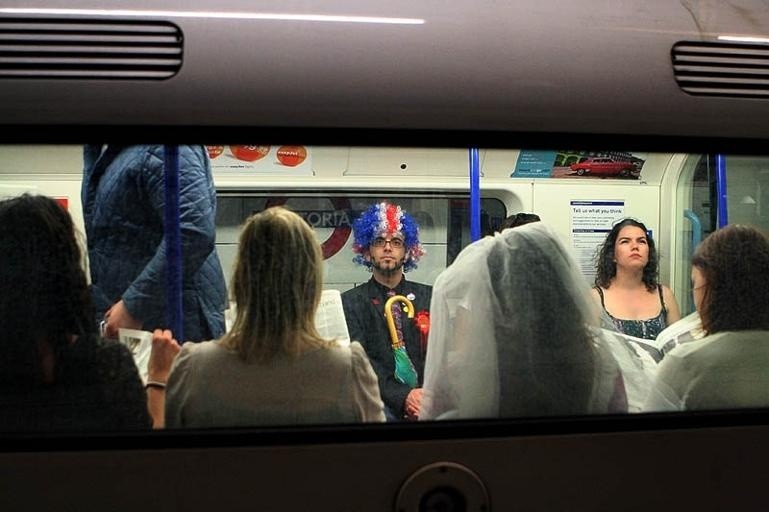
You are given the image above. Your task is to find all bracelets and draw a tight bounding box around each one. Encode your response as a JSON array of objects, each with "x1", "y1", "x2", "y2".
[{"x1": 143, "y1": 379, "x2": 168, "y2": 391}]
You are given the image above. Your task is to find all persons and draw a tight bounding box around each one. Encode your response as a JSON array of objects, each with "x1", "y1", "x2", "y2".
[
  {"x1": 415, "y1": 209, "x2": 629, "y2": 422},
  {"x1": 583, "y1": 214, "x2": 683, "y2": 371},
  {"x1": 339, "y1": 199, "x2": 459, "y2": 424},
  {"x1": 637, "y1": 222, "x2": 768, "y2": 414},
  {"x1": 78, "y1": 144, "x2": 231, "y2": 347},
  {"x1": 0, "y1": 190, "x2": 182, "y2": 438},
  {"x1": 497, "y1": 210, "x2": 543, "y2": 237},
  {"x1": 161, "y1": 203, "x2": 388, "y2": 430}
]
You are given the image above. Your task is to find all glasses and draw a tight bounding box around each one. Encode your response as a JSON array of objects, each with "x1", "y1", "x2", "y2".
[{"x1": 371, "y1": 235, "x2": 402, "y2": 250}]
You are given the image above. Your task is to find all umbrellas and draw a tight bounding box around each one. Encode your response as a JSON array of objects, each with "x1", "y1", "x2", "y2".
[{"x1": 383, "y1": 292, "x2": 421, "y2": 390}]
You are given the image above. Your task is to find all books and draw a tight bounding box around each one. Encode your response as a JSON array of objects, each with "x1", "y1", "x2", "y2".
[{"x1": 594, "y1": 305, "x2": 710, "y2": 412}]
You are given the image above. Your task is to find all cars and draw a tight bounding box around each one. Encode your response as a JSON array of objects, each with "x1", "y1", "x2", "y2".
[{"x1": 572, "y1": 158, "x2": 636, "y2": 177}]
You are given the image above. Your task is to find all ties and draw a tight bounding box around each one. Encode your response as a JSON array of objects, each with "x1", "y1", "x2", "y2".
[{"x1": 386, "y1": 290, "x2": 405, "y2": 348}]
